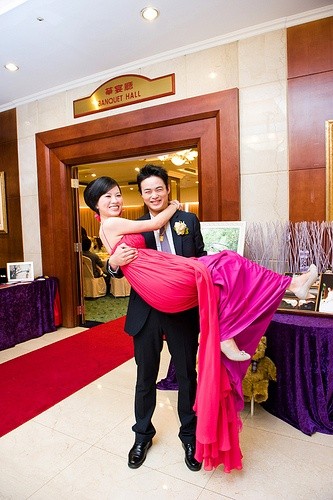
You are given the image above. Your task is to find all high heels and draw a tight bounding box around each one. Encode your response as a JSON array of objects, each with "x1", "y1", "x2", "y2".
[
  {"x1": 220, "y1": 338, "x2": 252, "y2": 361},
  {"x1": 293, "y1": 263, "x2": 318, "y2": 300}
]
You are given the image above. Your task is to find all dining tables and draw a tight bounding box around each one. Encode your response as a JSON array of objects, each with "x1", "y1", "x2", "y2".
[{"x1": 95, "y1": 253, "x2": 110, "y2": 272}]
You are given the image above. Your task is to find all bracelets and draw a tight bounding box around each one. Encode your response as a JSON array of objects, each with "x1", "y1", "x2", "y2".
[{"x1": 169, "y1": 201, "x2": 181, "y2": 210}]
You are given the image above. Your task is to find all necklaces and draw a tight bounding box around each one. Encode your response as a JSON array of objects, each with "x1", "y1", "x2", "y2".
[{"x1": 155, "y1": 223, "x2": 167, "y2": 242}]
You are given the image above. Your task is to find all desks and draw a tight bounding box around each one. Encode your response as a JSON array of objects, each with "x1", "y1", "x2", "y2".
[
  {"x1": 0, "y1": 276, "x2": 63, "y2": 352},
  {"x1": 154, "y1": 311, "x2": 333, "y2": 437}
]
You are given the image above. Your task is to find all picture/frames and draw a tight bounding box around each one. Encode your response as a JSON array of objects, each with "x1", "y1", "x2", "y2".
[
  {"x1": 275, "y1": 272, "x2": 333, "y2": 315},
  {"x1": 200, "y1": 221, "x2": 246, "y2": 258},
  {"x1": 7, "y1": 261, "x2": 35, "y2": 282}
]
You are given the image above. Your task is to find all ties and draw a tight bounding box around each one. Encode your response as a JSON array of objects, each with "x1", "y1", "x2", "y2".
[{"x1": 159, "y1": 227, "x2": 172, "y2": 253}]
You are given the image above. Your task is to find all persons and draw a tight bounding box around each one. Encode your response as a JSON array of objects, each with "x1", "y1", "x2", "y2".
[
  {"x1": 103, "y1": 165, "x2": 207, "y2": 472},
  {"x1": 82, "y1": 225, "x2": 109, "y2": 278},
  {"x1": 86, "y1": 179, "x2": 320, "y2": 362}
]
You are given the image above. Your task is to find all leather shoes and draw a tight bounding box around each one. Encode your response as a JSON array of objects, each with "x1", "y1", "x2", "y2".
[
  {"x1": 127, "y1": 437, "x2": 153, "y2": 469},
  {"x1": 182, "y1": 440, "x2": 203, "y2": 471}
]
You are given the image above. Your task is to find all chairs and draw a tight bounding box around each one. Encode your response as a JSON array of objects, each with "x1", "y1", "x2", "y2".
[
  {"x1": 109, "y1": 276, "x2": 131, "y2": 299},
  {"x1": 82, "y1": 256, "x2": 107, "y2": 298}
]
